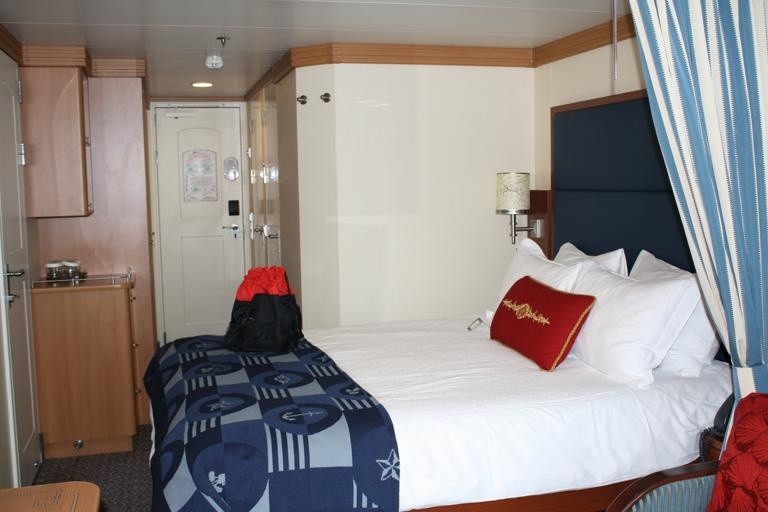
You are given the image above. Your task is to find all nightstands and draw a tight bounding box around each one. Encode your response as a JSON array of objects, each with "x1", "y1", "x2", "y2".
[{"x1": 699, "y1": 429, "x2": 725, "y2": 461}]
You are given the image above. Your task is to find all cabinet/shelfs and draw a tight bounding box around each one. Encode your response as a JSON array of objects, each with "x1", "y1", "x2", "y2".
[
  {"x1": 36, "y1": 75, "x2": 155, "y2": 426},
  {"x1": 18, "y1": 68, "x2": 93, "y2": 218},
  {"x1": 31, "y1": 283, "x2": 152, "y2": 457},
  {"x1": 247, "y1": 68, "x2": 299, "y2": 315}
]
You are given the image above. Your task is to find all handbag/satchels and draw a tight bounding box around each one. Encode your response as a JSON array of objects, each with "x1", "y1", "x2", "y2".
[{"x1": 223, "y1": 265, "x2": 302, "y2": 353}]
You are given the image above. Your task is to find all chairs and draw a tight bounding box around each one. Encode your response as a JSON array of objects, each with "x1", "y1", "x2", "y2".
[{"x1": 604, "y1": 456, "x2": 718, "y2": 512}]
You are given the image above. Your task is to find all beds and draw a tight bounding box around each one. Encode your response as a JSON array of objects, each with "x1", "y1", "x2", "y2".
[{"x1": 149, "y1": 236, "x2": 753, "y2": 512}]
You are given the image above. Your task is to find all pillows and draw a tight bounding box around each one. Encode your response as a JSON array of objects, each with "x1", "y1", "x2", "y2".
[
  {"x1": 493, "y1": 239, "x2": 579, "y2": 308},
  {"x1": 629, "y1": 247, "x2": 719, "y2": 377},
  {"x1": 552, "y1": 241, "x2": 693, "y2": 375},
  {"x1": 597, "y1": 248, "x2": 632, "y2": 279},
  {"x1": 490, "y1": 276, "x2": 594, "y2": 370}
]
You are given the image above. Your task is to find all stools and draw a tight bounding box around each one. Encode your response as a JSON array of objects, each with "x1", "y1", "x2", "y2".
[{"x1": 0, "y1": 480, "x2": 101, "y2": 512}]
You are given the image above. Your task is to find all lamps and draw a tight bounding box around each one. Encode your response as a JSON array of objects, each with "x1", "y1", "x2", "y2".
[
  {"x1": 205, "y1": 55, "x2": 224, "y2": 69},
  {"x1": 494, "y1": 173, "x2": 541, "y2": 244}
]
